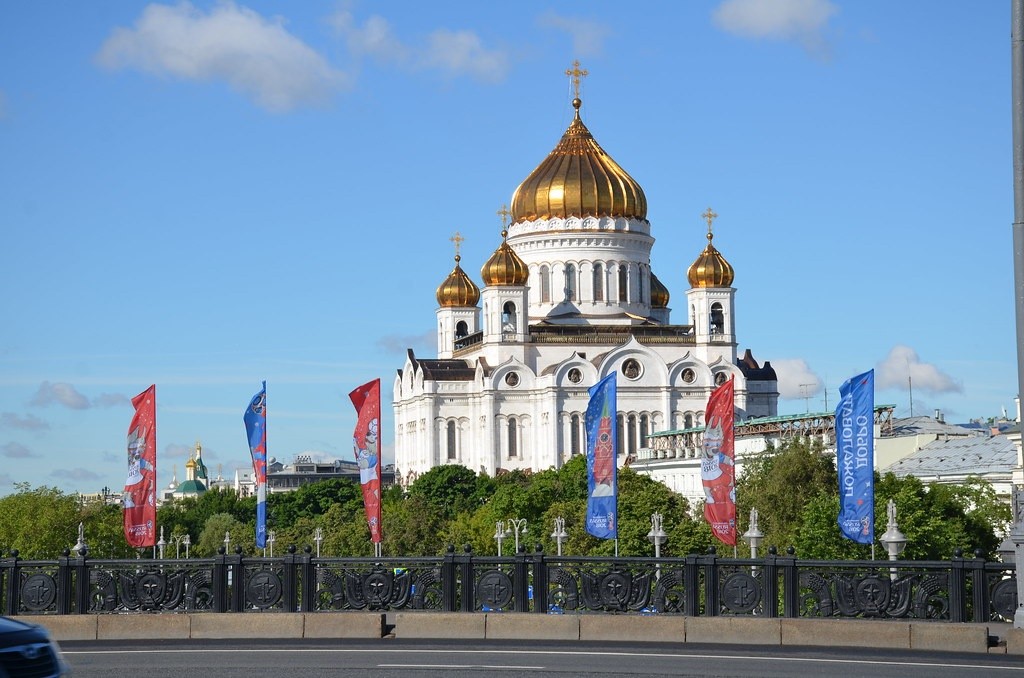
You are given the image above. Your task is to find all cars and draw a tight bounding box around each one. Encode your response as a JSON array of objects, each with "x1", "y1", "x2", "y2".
[{"x1": 0, "y1": 616, "x2": 69, "y2": 678}]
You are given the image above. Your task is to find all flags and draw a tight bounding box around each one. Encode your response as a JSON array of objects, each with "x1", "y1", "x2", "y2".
[
  {"x1": 585, "y1": 371, "x2": 620, "y2": 540},
  {"x1": 349, "y1": 377, "x2": 383, "y2": 543},
  {"x1": 700, "y1": 372, "x2": 735, "y2": 549},
  {"x1": 244, "y1": 379, "x2": 268, "y2": 548},
  {"x1": 124, "y1": 384, "x2": 157, "y2": 547},
  {"x1": 833, "y1": 367, "x2": 874, "y2": 546}
]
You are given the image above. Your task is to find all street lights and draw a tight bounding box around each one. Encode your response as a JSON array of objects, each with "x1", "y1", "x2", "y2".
[
  {"x1": 507, "y1": 517, "x2": 528, "y2": 553},
  {"x1": 314, "y1": 528, "x2": 324, "y2": 557},
  {"x1": 550, "y1": 516, "x2": 568, "y2": 606},
  {"x1": 157, "y1": 525, "x2": 166, "y2": 559},
  {"x1": 647, "y1": 510, "x2": 668, "y2": 580},
  {"x1": 743, "y1": 506, "x2": 765, "y2": 615},
  {"x1": 879, "y1": 499, "x2": 907, "y2": 580},
  {"x1": 224, "y1": 531, "x2": 230, "y2": 556},
  {"x1": 267, "y1": 530, "x2": 276, "y2": 558},
  {"x1": 170, "y1": 534, "x2": 186, "y2": 559},
  {"x1": 183, "y1": 534, "x2": 191, "y2": 559},
  {"x1": 492, "y1": 521, "x2": 506, "y2": 556},
  {"x1": 72, "y1": 522, "x2": 88, "y2": 559}
]
